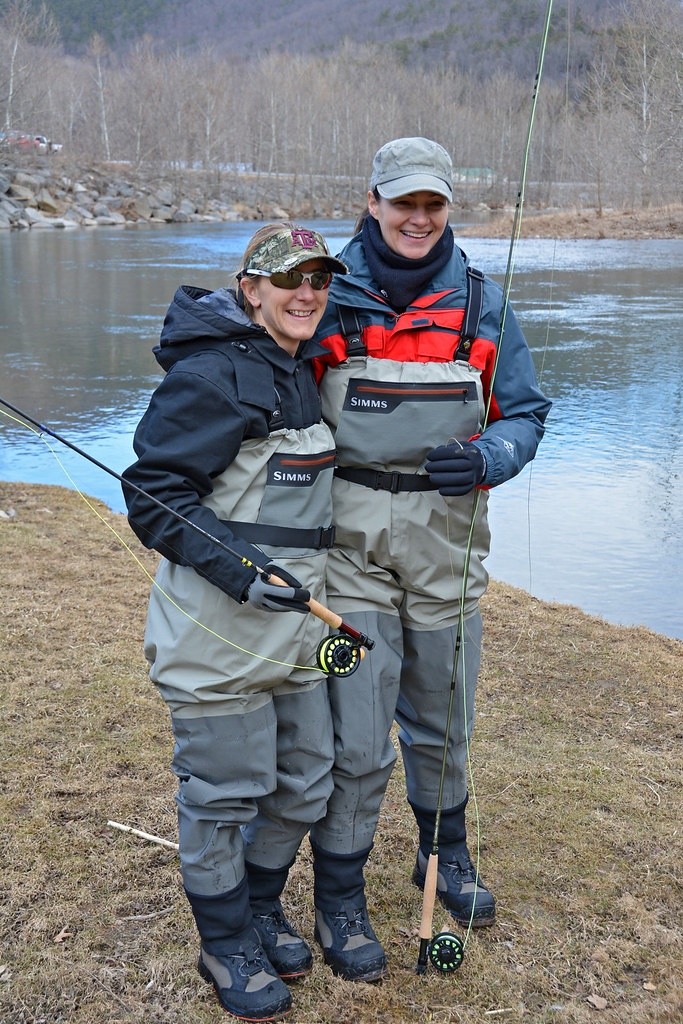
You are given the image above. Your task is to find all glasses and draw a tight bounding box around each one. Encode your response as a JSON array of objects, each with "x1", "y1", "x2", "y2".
[{"x1": 245, "y1": 268, "x2": 333, "y2": 290}]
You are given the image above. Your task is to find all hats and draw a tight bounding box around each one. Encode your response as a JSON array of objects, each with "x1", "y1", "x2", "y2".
[
  {"x1": 243, "y1": 229, "x2": 349, "y2": 276},
  {"x1": 371, "y1": 137, "x2": 454, "y2": 205}
]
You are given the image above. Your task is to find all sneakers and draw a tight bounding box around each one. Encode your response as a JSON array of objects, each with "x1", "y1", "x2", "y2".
[
  {"x1": 315, "y1": 902, "x2": 388, "y2": 983},
  {"x1": 197, "y1": 946, "x2": 292, "y2": 1021},
  {"x1": 412, "y1": 844, "x2": 496, "y2": 928},
  {"x1": 245, "y1": 893, "x2": 314, "y2": 982}
]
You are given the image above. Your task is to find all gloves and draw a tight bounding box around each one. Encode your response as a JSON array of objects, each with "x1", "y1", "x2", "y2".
[
  {"x1": 424, "y1": 440, "x2": 486, "y2": 498},
  {"x1": 246, "y1": 558, "x2": 310, "y2": 614}
]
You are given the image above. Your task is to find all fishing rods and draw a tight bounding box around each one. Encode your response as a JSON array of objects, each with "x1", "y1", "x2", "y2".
[
  {"x1": 0, "y1": 397, "x2": 376, "y2": 677},
  {"x1": 415, "y1": 1, "x2": 559, "y2": 975}
]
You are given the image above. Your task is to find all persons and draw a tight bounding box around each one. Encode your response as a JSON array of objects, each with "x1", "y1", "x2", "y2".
[
  {"x1": 310, "y1": 136, "x2": 553, "y2": 985},
  {"x1": 120, "y1": 223, "x2": 348, "y2": 1021}
]
